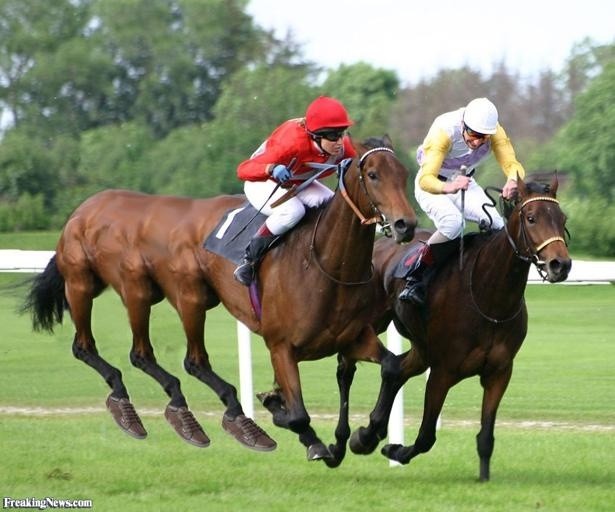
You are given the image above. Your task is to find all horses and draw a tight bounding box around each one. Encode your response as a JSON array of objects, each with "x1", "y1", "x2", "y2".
[
  {"x1": 23, "y1": 135, "x2": 419, "y2": 469},
  {"x1": 322, "y1": 170, "x2": 572, "y2": 484}
]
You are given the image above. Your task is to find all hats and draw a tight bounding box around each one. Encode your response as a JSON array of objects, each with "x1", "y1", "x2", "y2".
[
  {"x1": 306, "y1": 96, "x2": 354, "y2": 132},
  {"x1": 463, "y1": 98, "x2": 498, "y2": 135}
]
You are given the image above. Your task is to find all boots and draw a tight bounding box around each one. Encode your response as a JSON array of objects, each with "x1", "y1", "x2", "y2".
[
  {"x1": 398, "y1": 246, "x2": 442, "y2": 307},
  {"x1": 234, "y1": 221, "x2": 276, "y2": 286}
]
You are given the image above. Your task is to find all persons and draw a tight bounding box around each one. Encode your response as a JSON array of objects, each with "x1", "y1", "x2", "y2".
[
  {"x1": 235, "y1": 95, "x2": 356, "y2": 288},
  {"x1": 399, "y1": 97, "x2": 525, "y2": 301}
]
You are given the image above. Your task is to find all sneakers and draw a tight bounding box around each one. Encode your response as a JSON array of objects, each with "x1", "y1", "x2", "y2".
[
  {"x1": 163, "y1": 407, "x2": 211, "y2": 448},
  {"x1": 105, "y1": 395, "x2": 149, "y2": 438},
  {"x1": 221, "y1": 413, "x2": 278, "y2": 451}
]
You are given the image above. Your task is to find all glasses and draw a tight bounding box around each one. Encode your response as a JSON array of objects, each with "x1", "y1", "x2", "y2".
[
  {"x1": 464, "y1": 126, "x2": 487, "y2": 138},
  {"x1": 314, "y1": 130, "x2": 345, "y2": 141}
]
